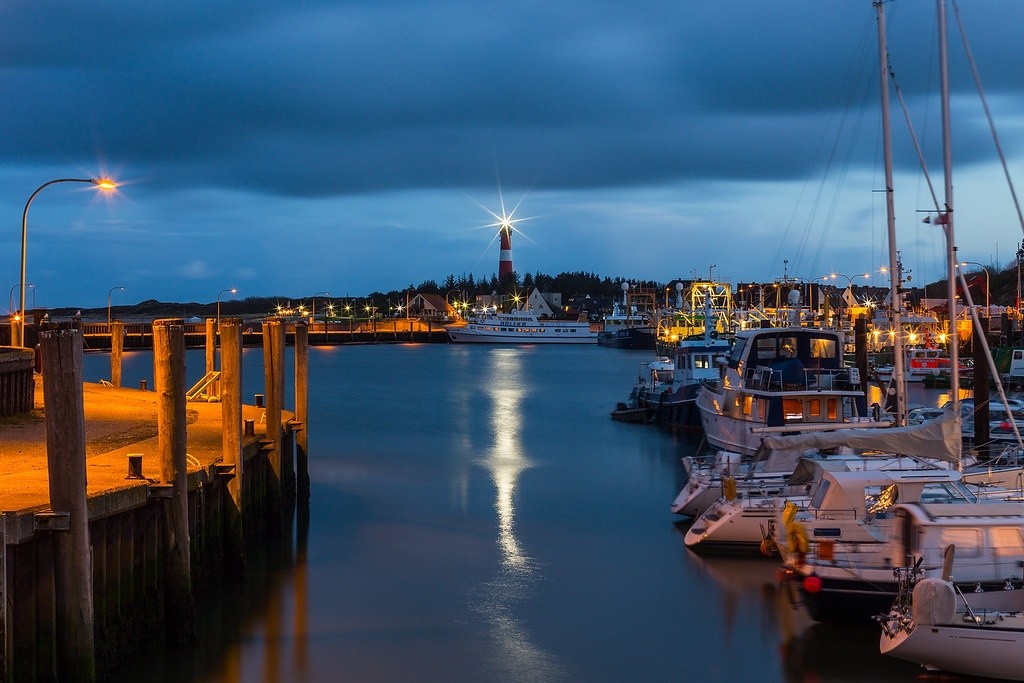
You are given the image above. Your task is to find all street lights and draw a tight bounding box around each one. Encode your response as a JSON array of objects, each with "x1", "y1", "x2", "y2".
[
  {"x1": 106, "y1": 287, "x2": 125, "y2": 333},
  {"x1": 750, "y1": 284, "x2": 776, "y2": 311},
  {"x1": 217, "y1": 289, "x2": 236, "y2": 333},
  {"x1": 830, "y1": 274, "x2": 868, "y2": 307},
  {"x1": 19, "y1": 176, "x2": 116, "y2": 349},
  {"x1": 792, "y1": 277, "x2": 827, "y2": 312},
  {"x1": 9, "y1": 283, "x2": 34, "y2": 324},
  {"x1": 739, "y1": 289, "x2": 753, "y2": 309},
  {"x1": 311, "y1": 291, "x2": 329, "y2": 331},
  {"x1": 406, "y1": 287, "x2": 416, "y2": 318},
  {"x1": 962, "y1": 261, "x2": 991, "y2": 317}
]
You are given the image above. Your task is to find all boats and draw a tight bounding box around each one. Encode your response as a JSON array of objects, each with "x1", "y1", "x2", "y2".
[
  {"x1": 449, "y1": 315, "x2": 599, "y2": 344},
  {"x1": 599, "y1": 0, "x2": 1024, "y2": 683}
]
